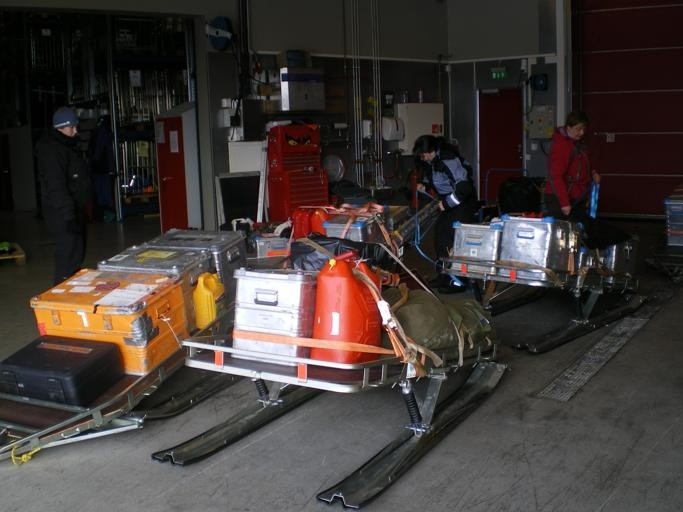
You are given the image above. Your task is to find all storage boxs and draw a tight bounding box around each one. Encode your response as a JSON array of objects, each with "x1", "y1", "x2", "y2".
[
  {"x1": 96, "y1": 224, "x2": 246, "y2": 332},
  {"x1": 662, "y1": 186, "x2": 683, "y2": 249},
  {"x1": 502, "y1": 213, "x2": 639, "y2": 290},
  {"x1": 29, "y1": 266, "x2": 187, "y2": 379},
  {"x1": 231, "y1": 260, "x2": 313, "y2": 363},
  {"x1": 0, "y1": 335, "x2": 123, "y2": 411},
  {"x1": 449, "y1": 218, "x2": 500, "y2": 277},
  {"x1": 251, "y1": 201, "x2": 409, "y2": 261}
]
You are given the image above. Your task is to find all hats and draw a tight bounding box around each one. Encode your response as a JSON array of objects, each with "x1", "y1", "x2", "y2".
[{"x1": 53, "y1": 107, "x2": 79, "y2": 129}]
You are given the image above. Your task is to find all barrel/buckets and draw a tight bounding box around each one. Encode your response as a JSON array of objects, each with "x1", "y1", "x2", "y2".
[
  {"x1": 191, "y1": 271, "x2": 227, "y2": 331},
  {"x1": 310, "y1": 251, "x2": 381, "y2": 365}
]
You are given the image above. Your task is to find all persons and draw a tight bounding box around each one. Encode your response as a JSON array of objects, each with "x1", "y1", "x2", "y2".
[
  {"x1": 412, "y1": 135, "x2": 480, "y2": 294},
  {"x1": 37, "y1": 107, "x2": 91, "y2": 285},
  {"x1": 544, "y1": 109, "x2": 601, "y2": 229}
]
